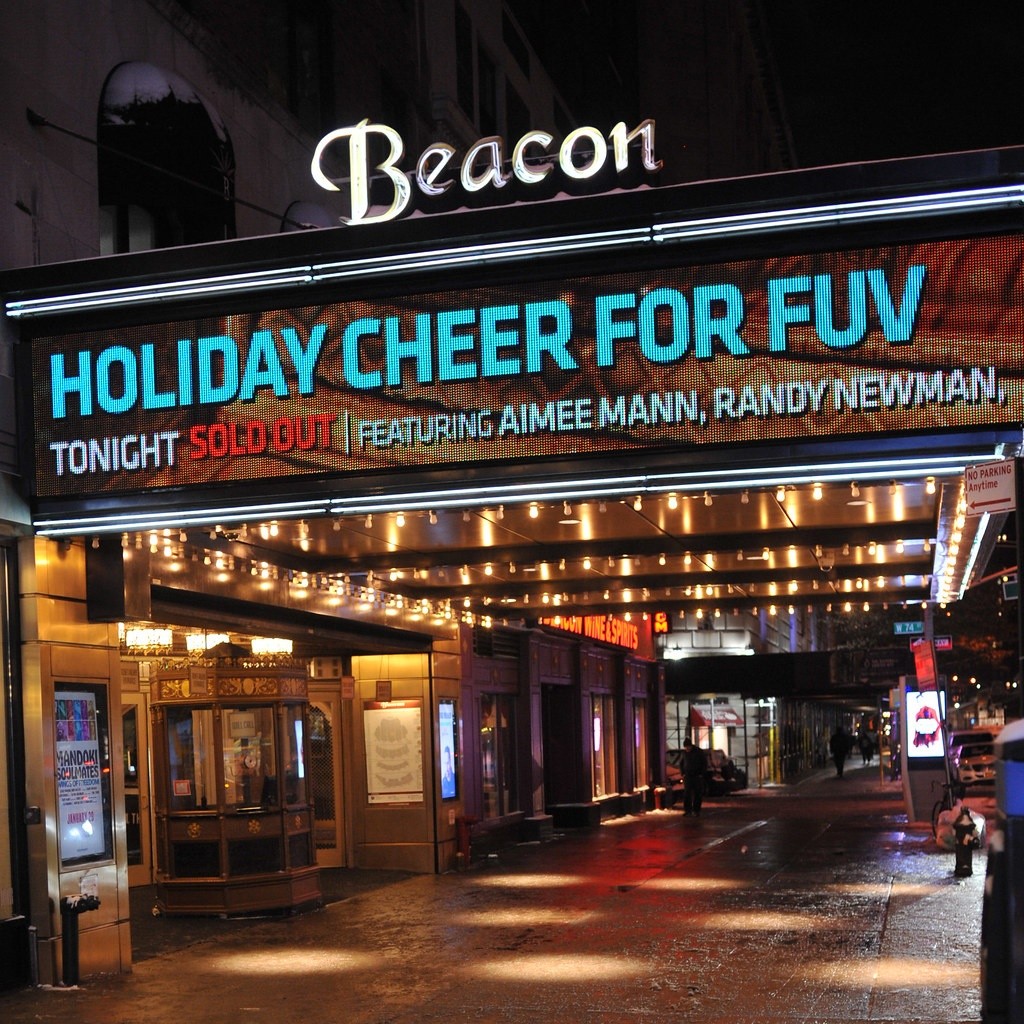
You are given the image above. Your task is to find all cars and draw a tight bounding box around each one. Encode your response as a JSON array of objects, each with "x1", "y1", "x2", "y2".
[
  {"x1": 947, "y1": 731, "x2": 993, "y2": 773},
  {"x1": 952, "y1": 742, "x2": 997, "y2": 783}
]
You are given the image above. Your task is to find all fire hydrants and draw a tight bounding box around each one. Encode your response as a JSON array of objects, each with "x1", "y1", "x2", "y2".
[{"x1": 952, "y1": 806, "x2": 980, "y2": 877}]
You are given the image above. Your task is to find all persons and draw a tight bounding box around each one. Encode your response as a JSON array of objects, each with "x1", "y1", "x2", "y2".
[
  {"x1": 55, "y1": 700, "x2": 95, "y2": 741},
  {"x1": 829, "y1": 727, "x2": 849, "y2": 776},
  {"x1": 442, "y1": 746, "x2": 455, "y2": 798},
  {"x1": 680, "y1": 740, "x2": 707, "y2": 816},
  {"x1": 848, "y1": 729, "x2": 879, "y2": 765},
  {"x1": 912, "y1": 694, "x2": 940, "y2": 748}
]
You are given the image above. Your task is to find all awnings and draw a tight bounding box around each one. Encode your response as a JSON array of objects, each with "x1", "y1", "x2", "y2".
[
  {"x1": 690, "y1": 706, "x2": 744, "y2": 726},
  {"x1": 100, "y1": 60, "x2": 237, "y2": 225}
]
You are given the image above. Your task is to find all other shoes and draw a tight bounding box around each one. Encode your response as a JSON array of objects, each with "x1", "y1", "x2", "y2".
[
  {"x1": 682, "y1": 811, "x2": 690, "y2": 817},
  {"x1": 691, "y1": 811, "x2": 699, "y2": 817}
]
